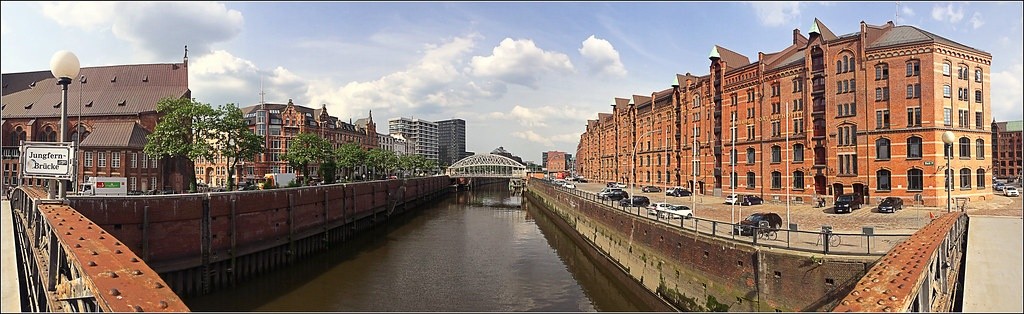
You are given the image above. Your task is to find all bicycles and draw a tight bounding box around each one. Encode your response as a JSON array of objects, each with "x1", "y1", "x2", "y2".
[
  {"x1": 815, "y1": 228, "x2": 842, "y2": 247},
  {"x1": 755, "y1": 221, "x2": 777, "y2": 241}
]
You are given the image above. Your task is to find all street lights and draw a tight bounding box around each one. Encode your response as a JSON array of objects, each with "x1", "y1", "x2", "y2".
[
  {"x1": 629, "y1": 129, "x2": 662, "y2": 207},
  {"x1": 942, "y1": 131, "x2": 956, "y2": 213},
  {"x1": 49, "y1": 49, "x2": 81, "y2": 197},
  {"x1": 571, "y1": 146, "x2": 578, "y2": 185},
  {"x1": 547, "y1": 154, "x2": 559, "y2": 181}
]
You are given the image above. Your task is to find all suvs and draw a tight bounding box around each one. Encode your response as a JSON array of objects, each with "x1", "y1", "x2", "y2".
[{"x1": 833, "y1": 193, "x2": 863, "y2": 214}]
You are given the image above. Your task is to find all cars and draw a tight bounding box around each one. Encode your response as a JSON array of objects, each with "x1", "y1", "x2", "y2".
[
  {"x1": 994, "y1": 182, "x2": 1006, "y2": 191},
  {"x1": 992, "y1": 174, "x2": 1014, "y2": 188},
  {"x1": 661, "y1": 204, "x2": 693, "y2": 219},
  {"x1": 596, "y1": 182, "x2": 630, "y2": 201},
  {"x1": 1003, "y1": 185, "x2": 1020, "y2": 197},
  {"x1": 620, "y1": 195, "x2": 650, "y2": 207},
  {"x1": 877, "y1": 196, "x2": 903, "y2": 213},
  {"x1": 741, "y1": 194, "x2": 763, "y2": 206},
  {"x1": 542, "y1": 173, "x2": 588, "y2": 190},
  {"x1": 648, "y1": 203, "x2": 673, "y2": 216},
  {"x1": 734, "y1": 212, "x2": 782, "y2": 236},
  {"x1": 725, "y1": 193, "x2": 739, "y2": 205},
  {"x1": 666, "y1": 186, "x2": 692, "y2": 197},
  {"x1": 642, "y1": 185, "x2": 662, "y2": 193}
]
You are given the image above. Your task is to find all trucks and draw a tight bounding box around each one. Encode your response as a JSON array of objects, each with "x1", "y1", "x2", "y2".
[{"x1": 77, "y1": 176, "x2": 128, "y2": 196}]
